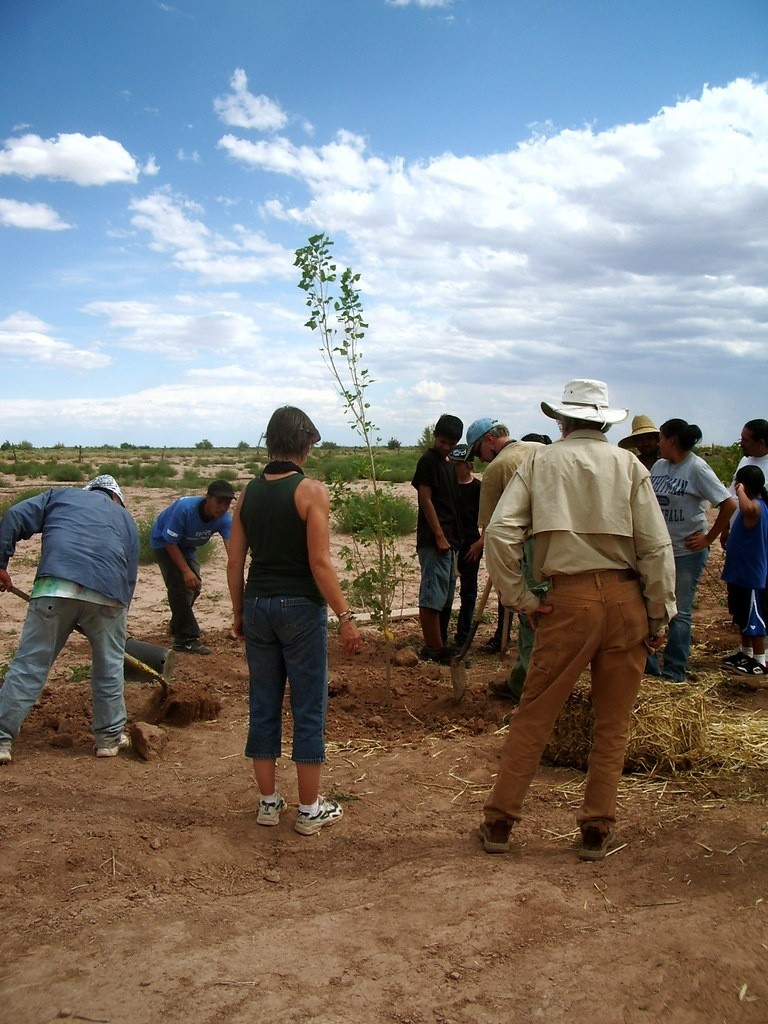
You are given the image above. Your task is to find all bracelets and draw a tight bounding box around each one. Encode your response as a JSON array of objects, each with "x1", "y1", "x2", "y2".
[{"x1": 336, "y1": 608, "x2": 354, "y2": 624}]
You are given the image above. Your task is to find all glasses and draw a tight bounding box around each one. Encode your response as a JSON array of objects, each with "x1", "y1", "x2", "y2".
[{"x1": 555, "y1": 414, "x2": 566, "y2": 425}]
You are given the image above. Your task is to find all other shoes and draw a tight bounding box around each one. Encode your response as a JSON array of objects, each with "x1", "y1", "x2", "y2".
[
  {"x1": 489, "y1": 678, "x2": 519, "y2": 704},
  {"x1": 419, "y1": 646, "x2": 471, "y2": 669},
  {"x1": 655, "y1": 675, "x2": 687, "y2": 686},
  {"x1": 642, "y1": 668, "x2": 661, "y2": 678},
  {"x1": 479, "y1": 639, "x2": 501, "y2": 653},
  {"x1": 452, "y1": 636, "x2": 470, "y2": 650}
]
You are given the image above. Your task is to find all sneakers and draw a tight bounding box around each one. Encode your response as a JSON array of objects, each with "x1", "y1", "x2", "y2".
[
  {"x1": 721, "y1": 651, "x2": 752, "y2": 669},
  {"x1": 257, "y1": 793, "x2": 288, "y2": 826},
  {"x1": 0, "y1": 736, "x2": 13, "y2": 761},
  {"x1": 96, "y1": 733, "x2": 129, "y2": 757},
  {"x1": 172, "y1": 637, "x2": 210, "y2": 655},
  {"x1": 733, "y1": 658, "x2": 768, "y2": 678},
  {"x1": 476, "y1": 818, "x2": 511, "y2": 853},
  {"x1": 294, "y1": 795, "x2": 343, "y2": 836},
  {"x1": 579, "y1": 819, "x2": 618, "y2": 859}
]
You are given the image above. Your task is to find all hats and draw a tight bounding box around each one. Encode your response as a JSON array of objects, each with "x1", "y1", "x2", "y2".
[
  {"x1": 208, "y1": 480, "x2": 238, "y2": 501},
  {"x1": 448, "y1": 444, "x2": 472, "y2": 462},
  {"x1": 617, "y1": 413, "x2": 661, "y2": 451},
  {"x1": 86, "y1": 474, "x2": 125, "y2": 506},
  {"x1": 540, "y1": 378, "x2": 629, "y2": 425},
  {"x1": 463, "y1": 417, "x2": 501, "y2": 462}
]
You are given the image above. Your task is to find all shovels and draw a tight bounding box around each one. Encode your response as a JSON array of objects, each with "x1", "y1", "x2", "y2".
[
  {"x1": 499, "y1": 607, "x2": 516, "y2": 663},
  {"x1": 449, "y1": 576, "x2": 492, "y2": 702},
  {"x1": 0, "y1": 580, "x2": 170, "y2": 691}
]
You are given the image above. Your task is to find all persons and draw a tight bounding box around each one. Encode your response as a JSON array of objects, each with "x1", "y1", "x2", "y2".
[
  {"x1": 0, "y1": 474, "x2": 138, "y2": 763},
  {"x1": 227, "y1": 405, "x2": 362, "y2": 836},
  {"x1": 412, "y1": 413, "x2": 768, "y2": 703},
  {"x1": 477, "y1": 379, "x2": 677, "y2": 862},
  {"x1": 149, "y1": 480, "x2": 237, "y2": 655}
]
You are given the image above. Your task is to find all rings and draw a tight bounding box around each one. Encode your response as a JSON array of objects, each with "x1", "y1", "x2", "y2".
[{"x1": 353, "y1": 645, "x2": 358, "y2": 648}]
